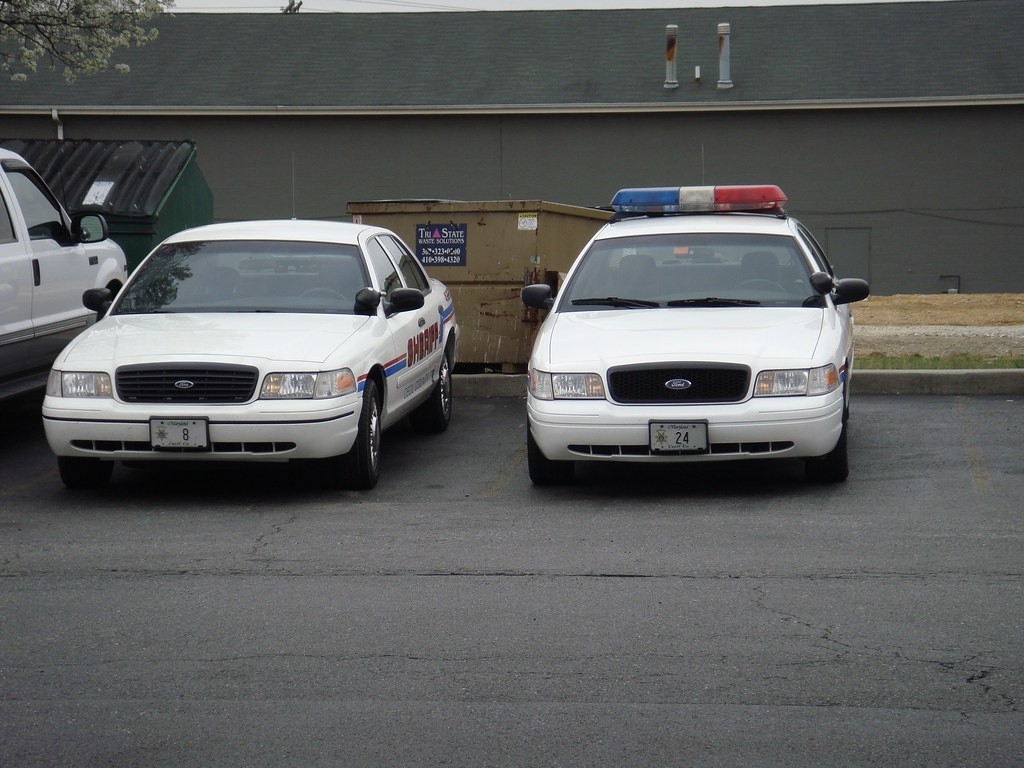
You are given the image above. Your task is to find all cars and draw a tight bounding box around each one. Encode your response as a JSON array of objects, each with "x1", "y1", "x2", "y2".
[
  {"x1": 39, "y1": 218, "x2": 462, "y2": 491},
  {"x1": 522, "y1": 183, "x2": 871, "y2": 490},
  {"x1": 0, "y1": 148, "x2": 129, "y2": 400}
]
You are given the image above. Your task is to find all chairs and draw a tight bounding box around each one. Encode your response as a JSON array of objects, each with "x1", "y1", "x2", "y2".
[
  {"x1": 619, "y1": 255, "x2": 656, "y2": 285},
  {"x1": 741, "y1": 252, "x2": 781, "y2": 281},
  {"x1": 317, "y1": 264, "x2": 360, "y2": 291},
  {"x1": 194, "y1": 266, "x2": 241, "y2": 301}
]
